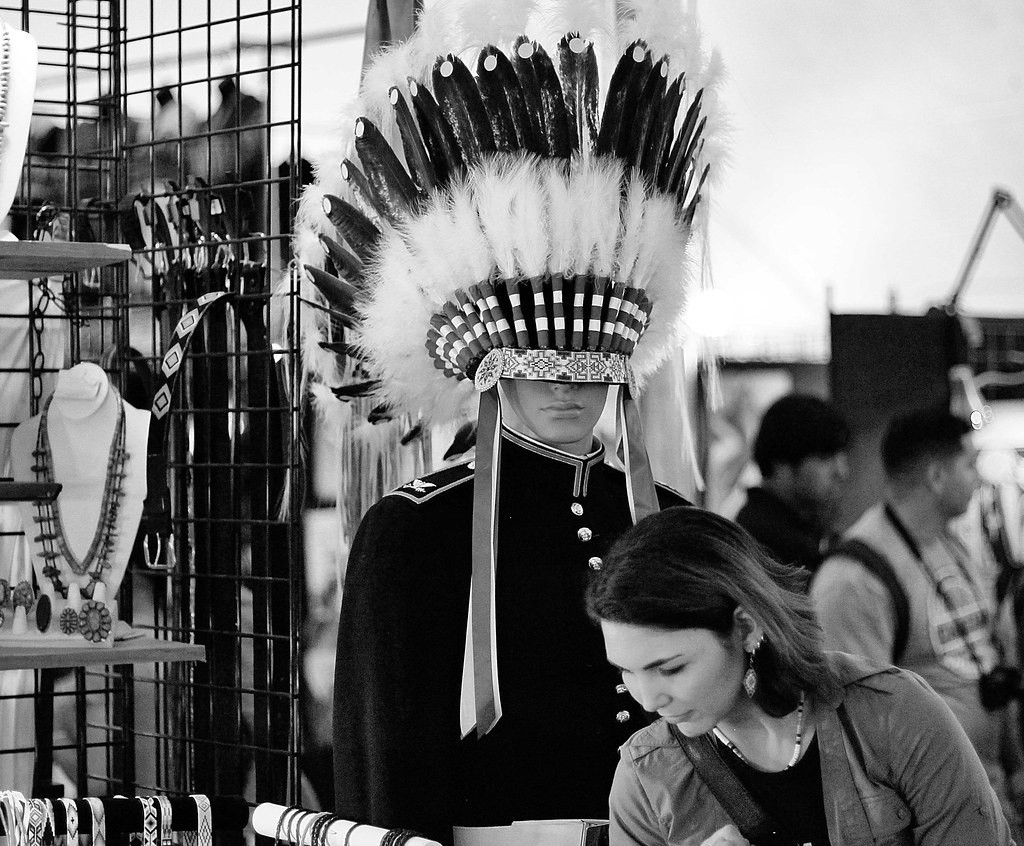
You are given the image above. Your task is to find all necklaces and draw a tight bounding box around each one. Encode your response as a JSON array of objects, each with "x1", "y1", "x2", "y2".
[
  {"x1": 712, "y1": 690, "x2": 805, "y2": 771},
  {"x1": 30, "y1": 384, "x2": 131, "y2": 600},
  {"x1": 0, "y1": 31, "x2": 9, "y2": 165}
]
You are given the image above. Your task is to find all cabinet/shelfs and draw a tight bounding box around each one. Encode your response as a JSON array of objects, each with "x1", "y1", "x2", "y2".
[{"x1": 1, "y1": 239, "x2": 206, "y2": 665}]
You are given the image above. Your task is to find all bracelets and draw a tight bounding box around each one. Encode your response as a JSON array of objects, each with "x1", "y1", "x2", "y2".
[{"x1": 276, "y1": 805, "x2": 422, "y2": 846}]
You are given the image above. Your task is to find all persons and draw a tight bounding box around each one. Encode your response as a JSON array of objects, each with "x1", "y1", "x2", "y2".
[
  {"x1": 586, "y1": 507, "x2": 1015, "y2": 845},
  {"x1": 736, "y1": 393, "x2": 850, "y2": 579},
  {"x1": 333, "y1": 377, "x2": 694, "y2": 846},
  {"x1": 801, "y1": 407, "x2": 1024, "y2": 830},
  {"x1": 75, "y1": 78, "x2": 264, "y2": 306}
]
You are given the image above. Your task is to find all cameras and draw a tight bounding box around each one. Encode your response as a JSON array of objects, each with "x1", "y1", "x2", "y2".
[{"x1": 978, "y1": 664, "x2": 1024, "y2": 717}]
